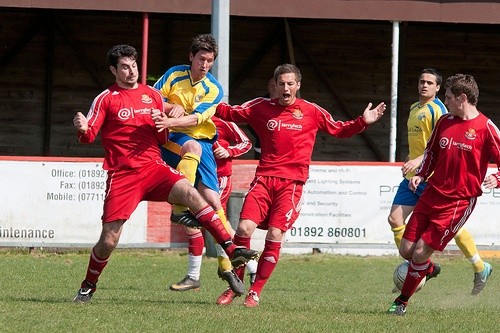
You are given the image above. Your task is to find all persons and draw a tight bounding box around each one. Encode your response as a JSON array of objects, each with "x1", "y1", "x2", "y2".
[
  {"x1": 216, "y1": 64, "x2": 387, "y2": 306},
  {"x1": 387, "y1": 73, "x2": 500, "y2": 316},
  {"x1": 170, "y1": 115, "x2": 252, "y2": 291},
  {"x1": 153, "y1": 34, "x2": 258, "y2": 296},
  {"x1": 388, "y1": 69, "x2": 492, "y2": 296},
  {"x1": 254, "y1": 78, "x2": 277, "y2": 160},
  {"x1": 395, "y1": 123, "x2": 409, "y2": 162},
  {"x1": 73, "y1": 45, "x2": 259, "y2": 306}
]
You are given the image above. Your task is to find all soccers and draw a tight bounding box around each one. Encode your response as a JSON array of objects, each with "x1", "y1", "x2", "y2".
[{"x1": 394, "y1": 262, "x2": 428, "y2": 293}]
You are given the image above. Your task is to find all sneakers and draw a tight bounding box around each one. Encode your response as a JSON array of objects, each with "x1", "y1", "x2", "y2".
[
  {"x1": 229, "y1": 245, "x2": 260, "y2": 269},
  {"x1": 386, "y1": 299, "x2": 408, "y2": 317},
  {"x1": 73, "y1": 284, "x2": 94, "y2": 306},
  {"x1": 169, "y1": 209, "x2": 203, "y2": 230},
  {"x1": 424, "y1": 259, "x2": 442, "y2": 283},
  {"x1": 470, "y1": 260, "x2": 494, "y2": 296},
  {"x1": 216, "y1": 266, "x2": 247, "y2": 295},
  {"x1": 215, "y1": 287, "x2": 237, "y2": 306},
  {"x1": 242, "y1": 290, "x2": 261, "y2": 310},
  {"x1": 170, "y1": 274, "x2": 201, "y2": 292}
]
